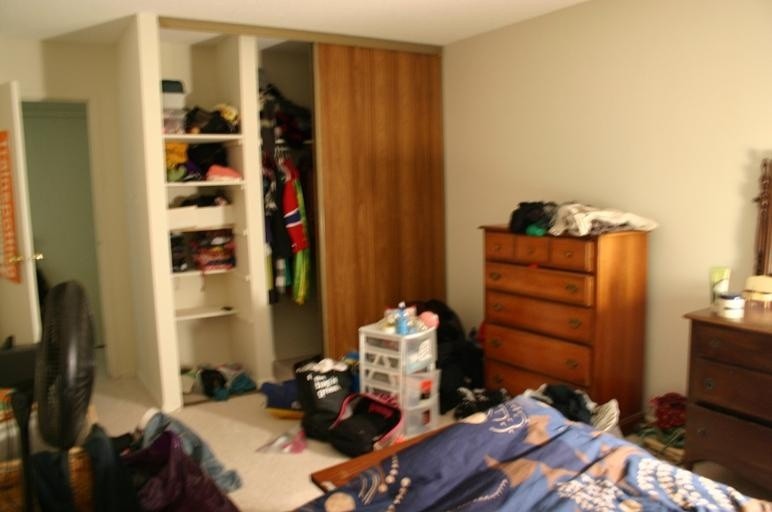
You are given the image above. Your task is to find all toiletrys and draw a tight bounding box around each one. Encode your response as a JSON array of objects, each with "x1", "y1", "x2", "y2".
[
  {"x1": 708, "y1": 266, "x2": 730, "y2": 313},
  {"x1": 718, "y1": 292, "x2": 745, "y2": 320}
]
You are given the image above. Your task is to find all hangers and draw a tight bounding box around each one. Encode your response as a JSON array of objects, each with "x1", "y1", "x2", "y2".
[{"x1": 273, "y1": 139, "x2": 294, "y2": 167}]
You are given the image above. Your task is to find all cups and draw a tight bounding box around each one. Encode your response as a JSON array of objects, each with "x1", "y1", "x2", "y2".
[{"x1": 706, "y1": 264, "x2": 732, "y2": 312}]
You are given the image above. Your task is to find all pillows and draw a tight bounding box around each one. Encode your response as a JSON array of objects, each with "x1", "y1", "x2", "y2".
[
  {"x1": 104, "y1": 428, "x2": 241, "y2": 512},
  {"x1": 132, "y1": 406, "x2": 242, "y2": 494}
]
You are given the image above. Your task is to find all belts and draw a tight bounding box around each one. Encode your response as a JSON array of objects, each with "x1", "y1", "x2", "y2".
[{"x1": 135, "y1": 408, "x2": 159, "y2": 440}]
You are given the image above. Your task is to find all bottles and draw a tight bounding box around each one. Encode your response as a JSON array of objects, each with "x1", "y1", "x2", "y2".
[{"x1": 395, "y1": 302, "x2": 408, "y2": 336}]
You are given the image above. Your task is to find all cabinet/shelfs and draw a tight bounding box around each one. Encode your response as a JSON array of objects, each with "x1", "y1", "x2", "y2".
[
  {"x1": 249, "y1": 24, "x2": 447, "y2": 390},
  {"x1": 358, "y1": 313, "x2": 442, "y2": 438},
  {"x1": 479, "y1": 225, "x2": 651, "y2": 435},
  {"x1": 114, "y1": 13, "x2": 272, "y2": 412},
  {"x1": 680, "y1": 293, "x2": 772, "y2": 493}
]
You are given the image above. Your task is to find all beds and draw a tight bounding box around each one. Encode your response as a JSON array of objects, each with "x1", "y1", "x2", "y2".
[{"x1": 306, "y1": 397, "x2": 771, "y2": 512}]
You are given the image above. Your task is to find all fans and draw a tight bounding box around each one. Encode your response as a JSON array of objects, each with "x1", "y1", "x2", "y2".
[{"x1": 0, "y1": 278, "x2": 95, "y2": 512}]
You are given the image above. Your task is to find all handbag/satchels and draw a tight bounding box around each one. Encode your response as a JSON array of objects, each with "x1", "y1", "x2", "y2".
[{"x1": 294, "y1": 352, "x2": 358, "y2": 439}]
